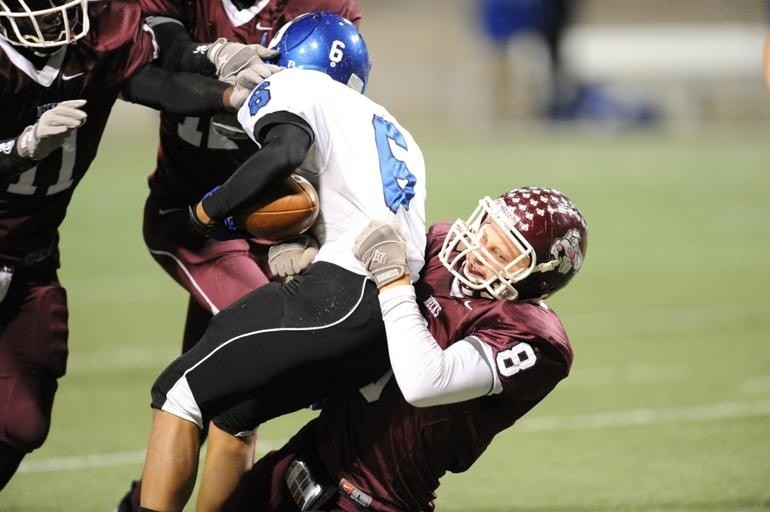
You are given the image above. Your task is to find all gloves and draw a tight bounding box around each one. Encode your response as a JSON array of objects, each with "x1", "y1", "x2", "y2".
[
  {"x1": 175, "y1": 204, "x2": 208, "y2": 249},
  {"x1": 268, "y1": 237, "x2": 319, "y2": 277},
  {"x1": 208, "y1": 38, "x2": 283, "y2": 108},
  {"x1": 353, "y1": 220, "x2": 409, "y2": 291},
  {"x1": 16, "y1": 100, "x2": 87, "y2": 159}
]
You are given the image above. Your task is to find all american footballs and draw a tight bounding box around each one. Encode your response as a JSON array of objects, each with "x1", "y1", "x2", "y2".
[{"x1": 232, "y1": 173, "x2": 320, "y2": 242}]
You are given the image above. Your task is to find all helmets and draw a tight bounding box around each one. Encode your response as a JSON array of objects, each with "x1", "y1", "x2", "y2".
[
  {"x1": 438, "y1": 185, "x2": 588, "y2": 302},
  {"x1": 265, "y1": 13, "x2": 369, "y2": 94},
  {"x1": 1, "y1": 2, "x2": 89, "y2": 52}
]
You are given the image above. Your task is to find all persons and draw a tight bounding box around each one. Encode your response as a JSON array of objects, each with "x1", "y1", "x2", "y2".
[
  {"x1": 208, "y1": 183, "x2": 588, "y2": 510},
  {"x1": 111, "y1": 9, "x2": 430, "y2": 511},
  {"x1": 1, "y1": 0, "x2": 285, "y2": 492},
  {"x1": 121, "y1": 1, "x2": 363, "y2": 511}
]
